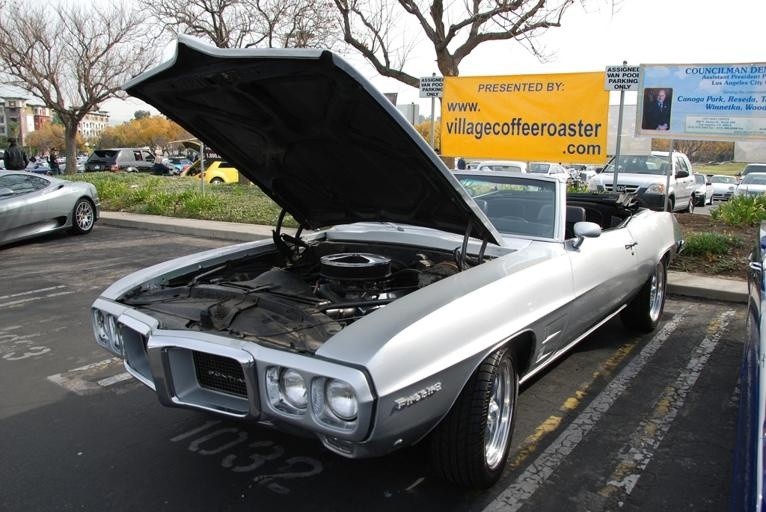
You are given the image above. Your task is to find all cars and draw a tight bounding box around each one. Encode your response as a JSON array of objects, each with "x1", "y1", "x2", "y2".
[
  {"x1": 0, "y1": 143, "x2": 257, "y2": 186},
  {"x1": 728, "y1": 216, "x2": 765, "y2": 512}
]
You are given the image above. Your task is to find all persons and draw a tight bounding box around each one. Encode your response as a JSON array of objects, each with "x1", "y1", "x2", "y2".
[
  {"x1": 154, "y1": 154, "x2": 162, "y2": 176},
  {"x1": 4, "y1": 138, "x2": 30, "y2": 170},
  {"x1": 642, "y1": 89, "x2": 671, "y2": 129},
  {"x1": 50, "y1": 147, "x2": 65, "y2": 174}
]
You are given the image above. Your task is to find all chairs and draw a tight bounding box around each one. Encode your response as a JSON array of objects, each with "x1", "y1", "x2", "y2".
[
  {"x1": 622, "y1": 162, "x2": 672, "y2": 174},
  {"x1": 484, "y1": 198, "x2": 620, "y2": 237}
]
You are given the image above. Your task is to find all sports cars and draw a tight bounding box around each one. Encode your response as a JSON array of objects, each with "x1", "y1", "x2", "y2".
[
  {"x1": 89, "y1": 24, "x2": 678, "y2": 494},
  {"x1": 0, "y1": 170, "x2": 102, "y2": 248}
]
